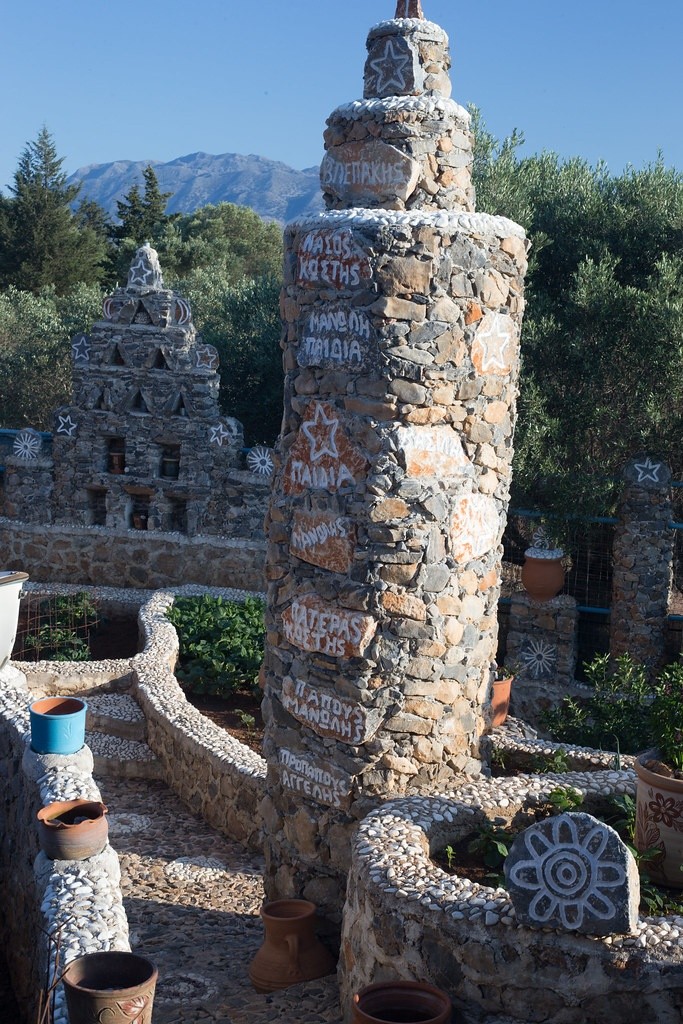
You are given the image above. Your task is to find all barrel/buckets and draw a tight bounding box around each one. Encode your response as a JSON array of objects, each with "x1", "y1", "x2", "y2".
[
  {"x1": 353, "y1": 980, "x2": 452, "y2": 1024},
  {"x1": 38, "y1": 799, "x2": 108, "y2": 861},
  {"x1": 29, "y1": 697, "x2": 87, "y2": 755},
  {"x1": 62, "y1": 951, "x2": 158, "y2": 1024}
]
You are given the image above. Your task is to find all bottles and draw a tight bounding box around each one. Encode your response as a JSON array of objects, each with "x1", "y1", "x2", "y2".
[{"x1": 247, "y1": 899, "x2": 329, "y2": 991}]
